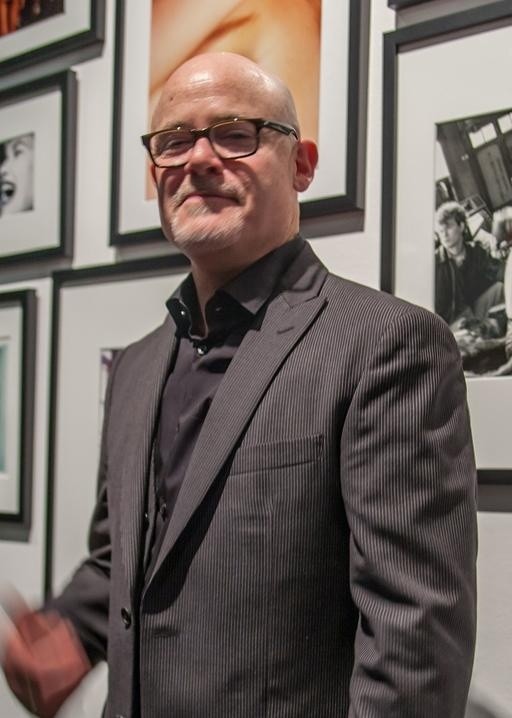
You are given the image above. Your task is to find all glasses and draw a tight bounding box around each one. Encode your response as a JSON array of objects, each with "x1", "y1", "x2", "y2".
[{"x1": 138, "y1": 113, "x2": 300, "y2": 168}]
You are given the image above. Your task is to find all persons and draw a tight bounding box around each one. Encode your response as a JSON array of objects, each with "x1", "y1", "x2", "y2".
[
  {"x1": 0, "y1": 53, "x2": 481, "y2": 718},
  {"x1": 0, "y1": 131, "x2": 33, "y2": 211},
  {"x1": 434, "y1": 198, "x2": 506, "y2": 326}
]
